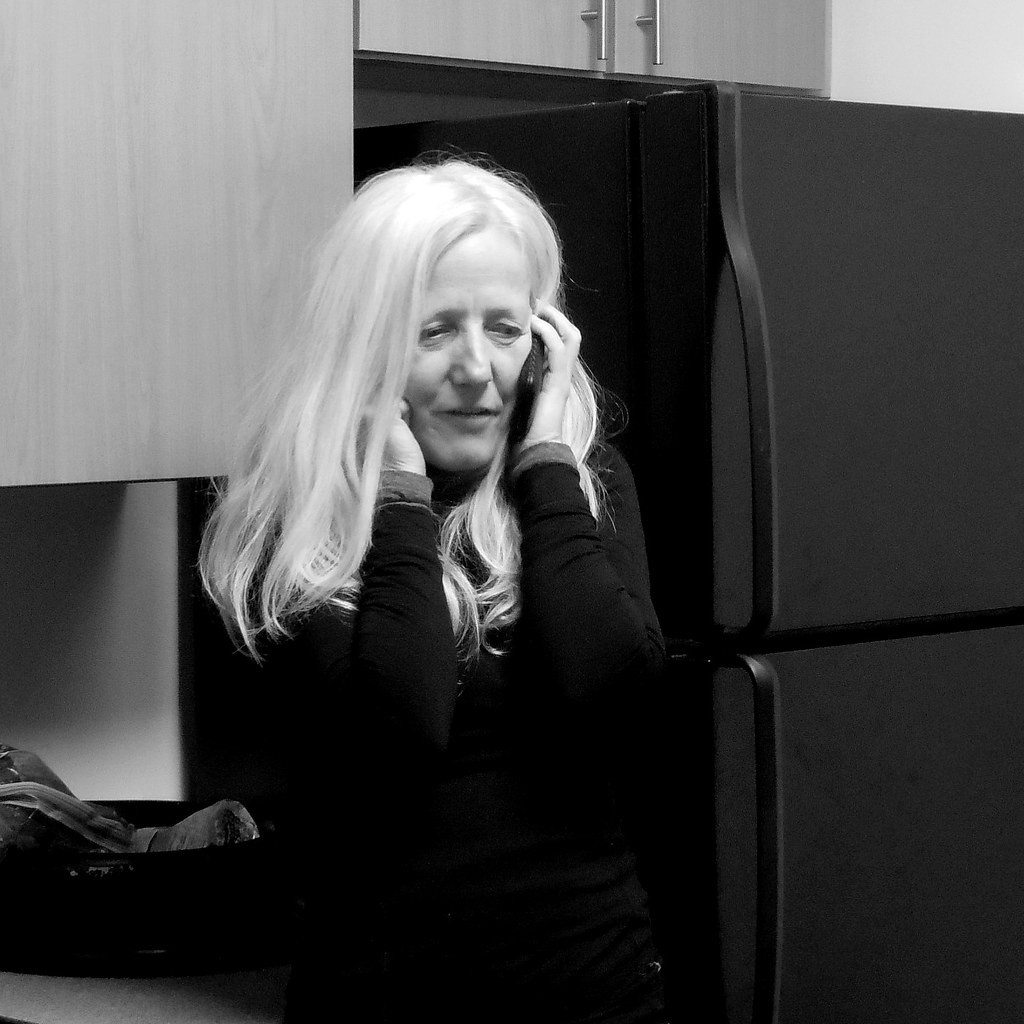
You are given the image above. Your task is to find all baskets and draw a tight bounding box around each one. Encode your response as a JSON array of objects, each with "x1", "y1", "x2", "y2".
[{"x1": 0, "y1": 801, "x2": 272, "y2": 977}]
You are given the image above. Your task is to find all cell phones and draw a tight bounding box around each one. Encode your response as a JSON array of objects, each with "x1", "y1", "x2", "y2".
[{"x1": 511, "y1": 332, "x2": 544, "y2": 441}]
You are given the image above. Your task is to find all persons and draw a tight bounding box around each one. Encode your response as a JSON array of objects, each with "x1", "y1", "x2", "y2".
[{"x1": 194, "y1": 155, "x2": 670, "y2": 1023}]
